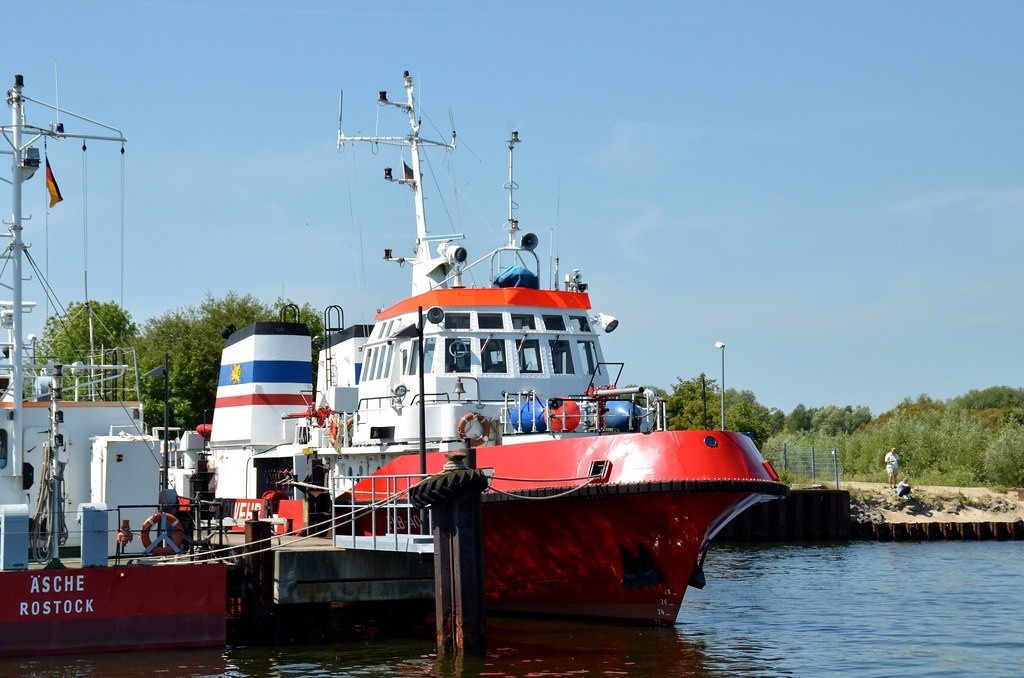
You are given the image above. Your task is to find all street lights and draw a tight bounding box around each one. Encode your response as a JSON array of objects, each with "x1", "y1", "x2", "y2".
[
  {"x1": 715, "y1": 341, "x2": 725, "y2": 433},
  {"x1": 27, "y1": 333, "x2": 36, "y2": 397}
]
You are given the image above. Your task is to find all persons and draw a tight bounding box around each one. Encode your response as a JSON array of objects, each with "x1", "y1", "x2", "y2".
[
  {"x1": 897, "y1": 478, "x2": 912, "y2": 499},
  {"x1": 885, "y1": 448, "x2": 901, "y2": 489}
]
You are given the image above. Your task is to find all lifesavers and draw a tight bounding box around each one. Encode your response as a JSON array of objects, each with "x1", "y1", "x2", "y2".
[
  {"x1": 329, "y1": 416, "x2": 337, "y2": 445},
  {"x1": 457, "y1": 414, "x2": 491, "y2": 449},
  {"x1": 139, "y1": 513, "x2": 185, "y2": 556},
  {"x1": 348, "y1": 417, "x2": 353, "y2": 446}
]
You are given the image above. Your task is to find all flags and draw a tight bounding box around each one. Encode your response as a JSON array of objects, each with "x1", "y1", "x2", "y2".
[
  {"x1": 46, "y1": 156, "x2": 64, "y2": 208},
  {"x1": 403, "y1": 160, "x2": 424, "y2": 189}
]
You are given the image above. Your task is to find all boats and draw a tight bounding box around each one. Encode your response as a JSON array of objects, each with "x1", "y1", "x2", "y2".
[{"x1": 0, "y1": 69, "x2": 792, "y2": 668}]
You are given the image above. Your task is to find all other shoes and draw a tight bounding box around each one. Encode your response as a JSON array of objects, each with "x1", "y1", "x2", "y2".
[
  {"x1": 903, "y1": 495, "x2": 908, "y2": 499},
  {"x1": 907, "y1": 494, "x2": 911, "y2": 499}
]
